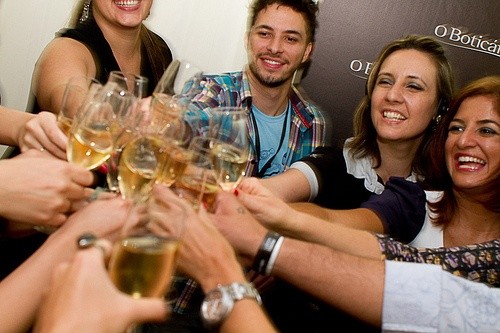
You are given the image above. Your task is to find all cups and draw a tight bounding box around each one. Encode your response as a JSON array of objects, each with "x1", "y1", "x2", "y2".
[{"x1": 55, "y1": 70, "x2": 250, "y2": 333}]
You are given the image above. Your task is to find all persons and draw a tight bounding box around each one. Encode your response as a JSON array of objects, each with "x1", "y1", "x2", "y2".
[
  {"x1": 0, "y1": 0, "x2": 174, "y2": 162},
  {"x1": 0, "y1": 105, "x2": 170, "y2": 333},
  {"x1": 125, "y1": 0, "x2": 326, "y2": 333},
  {"x1": 260, "y1": 33, "x2": 454, "y2": 208},
  {"x1": 286, "y1": 75, "x2": 500, "y2": 249},
  {"x1": 147, "y1": 176, "x2": 500, "y2": 333}
]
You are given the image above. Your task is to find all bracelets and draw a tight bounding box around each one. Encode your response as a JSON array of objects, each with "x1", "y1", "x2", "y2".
[{"x1": 251, "y1": 230, "x2": 284, "y2": 277}]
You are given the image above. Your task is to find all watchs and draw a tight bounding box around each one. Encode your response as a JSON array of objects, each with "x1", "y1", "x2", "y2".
[{"x1": 200, "y1": 282, "x2": 262, "y2": 329}]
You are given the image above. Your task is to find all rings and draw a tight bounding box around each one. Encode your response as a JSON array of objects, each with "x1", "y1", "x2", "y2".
[
  {"x1": 40, "y1": 147, "x2": 45, "y2": 152},
  {"x1": 76, "y1": 233, "x2": 110, "y2": 255}
]
